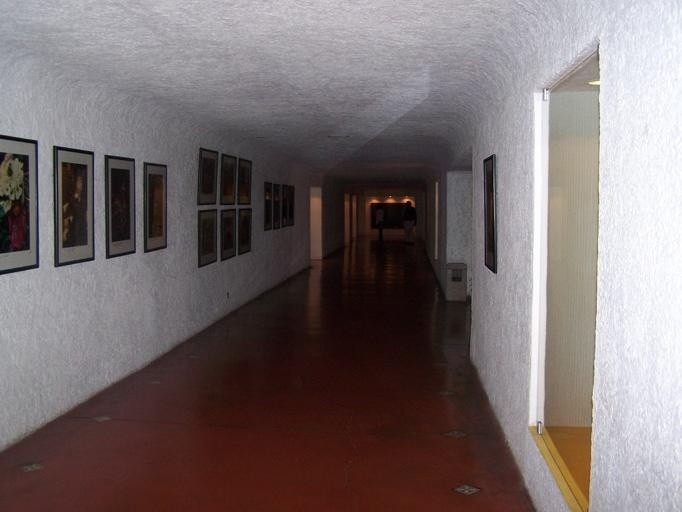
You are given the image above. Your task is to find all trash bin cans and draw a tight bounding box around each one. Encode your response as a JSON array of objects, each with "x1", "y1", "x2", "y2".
[{"x1": 445, "y1": 263, "x2": 467, "y2": 301}]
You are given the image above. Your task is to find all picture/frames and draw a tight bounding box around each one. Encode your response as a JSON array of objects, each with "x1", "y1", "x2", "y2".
[
  {"x1": 196, "y1": 208, "x2": 218, "y2": 268},
  {"x1": 238, "y1": 157, "x2": 252, "y2": 206},
  {"x1": 0, "y1": 134, "x2": 43, "y2": 276},
  {"x1": 197, "y1": 145, "x2": 219, "y2": 207},
  {"x1": 141, "y1": 159, "x2": 169, "y2": 253},
  {"x1": 219, "y1": 152, "x2": 237, "y2": 206},
  {"x1": 219, "y1": 208, "x2": 236, "y2": 262},
  {"x1": 237, "y1": 207, "x2": 252, "y2": 255},
  {"x1": 262, "y1": 180, "x2": 294, "y2": 231},
  {"x1": 50, "y1": 144, "x2": 96, "y2": 269},
  {"x1": 483, "y1": 150, "x2": 497, "y2": 276},
  {"x1": 102, "y1": 153, "x2": 137, "y2": 259}
]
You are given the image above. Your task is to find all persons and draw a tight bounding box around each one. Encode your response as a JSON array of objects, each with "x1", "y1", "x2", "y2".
[{"x1": 403, "y1": 201, "x2": 418, "y2": 244}]
